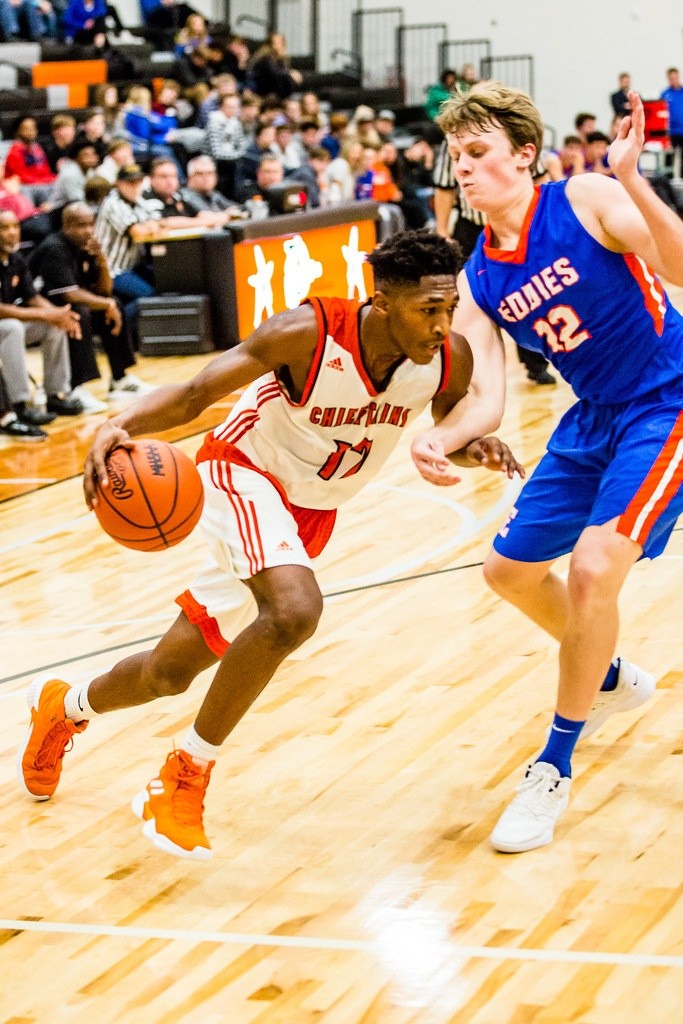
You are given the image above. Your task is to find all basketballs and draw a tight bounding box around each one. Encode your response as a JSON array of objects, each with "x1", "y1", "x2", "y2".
[{"x1": 92, "y1": 437, "x2": 205, "y2": 552}]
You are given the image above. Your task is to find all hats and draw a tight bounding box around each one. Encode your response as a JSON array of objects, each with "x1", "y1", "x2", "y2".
[
  {"x1": 117, "y1": 165, "x2": 143, "y2": 182},
  {"x1": 379, "y1": 109, "x2": 394, "y2": 121}
]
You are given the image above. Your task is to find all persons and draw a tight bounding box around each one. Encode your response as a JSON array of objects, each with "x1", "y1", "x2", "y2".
[
  {"x1": 0, "y1": 1, "x2": 683, "y2": 442},
  {"x1": 21, "y1": 229, "x2": 530, "y2": 861},
  {"x1": 408, "y1": 82, "x2": 683, "y2": 857}
]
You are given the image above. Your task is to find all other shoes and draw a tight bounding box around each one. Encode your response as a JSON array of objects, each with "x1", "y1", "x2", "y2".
[
  {"x1": 47, "y1": 394, "x2": 85, "y2": 416},
  {"x1": 13, "y1": 408, "x2": 58, "y2": 425}
]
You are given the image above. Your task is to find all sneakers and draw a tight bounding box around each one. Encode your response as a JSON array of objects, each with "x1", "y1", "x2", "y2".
[
  {"x1": 130, "y1": 749, "x2": 215, "y2": 861},
  {"x1": 0, "y1": 420, "x2": 46, "y2": 442},
  {"x1": 546, "y1": 656, "x2": 655, "y2": 745},
  {"x1": 527, "y1": 369, "x2": 556, "y2": 384},
  {"x1": 490, "y1": 763, "x2": 574, "y2": 852},
  {"x1": 68, "y1": 385, "x2": 108, "y2": 414},
  {"x1": 106, "y1": 371, "x2": 158, "y2": 400},
  {"x1": 17, "y1": 676, "x2": 89, "y2": 800}
]
